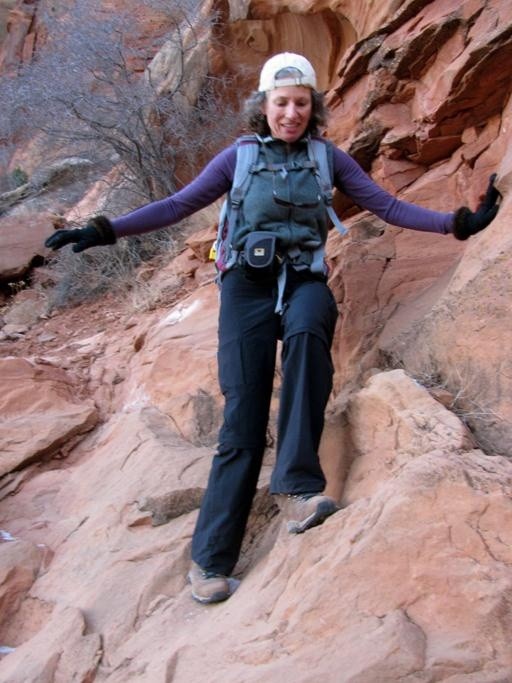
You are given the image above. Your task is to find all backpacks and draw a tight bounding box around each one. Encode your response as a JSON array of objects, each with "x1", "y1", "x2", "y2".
[{"x1": 210, "y1": 135, "x2": 349, "y2": 288}]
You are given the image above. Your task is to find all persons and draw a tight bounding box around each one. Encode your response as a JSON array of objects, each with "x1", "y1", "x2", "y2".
[{"x1": 45, "y1": 53, "x2": 504, "y2": 603}]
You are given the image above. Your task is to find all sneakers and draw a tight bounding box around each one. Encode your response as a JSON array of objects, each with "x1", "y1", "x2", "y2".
[
  {"x1": 275, "y1": 491, "x2": 338, "y2": 534},
  {"x1": 188, "y1": 559, "x2": 233, "y2": 605}
]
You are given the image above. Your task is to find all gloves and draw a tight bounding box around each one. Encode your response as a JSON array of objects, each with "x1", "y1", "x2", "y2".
[
  {"x1": 455, "y1": 173, "x2": 504, "y2": 239},
  {"x1": 44, "y1": 215, "x2": 117, "y2": 253}
]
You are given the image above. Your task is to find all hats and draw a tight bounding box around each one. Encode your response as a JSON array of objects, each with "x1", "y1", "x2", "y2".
[{"x1": 258, "y1": 50, "x2": 317, "y2": 93}]
